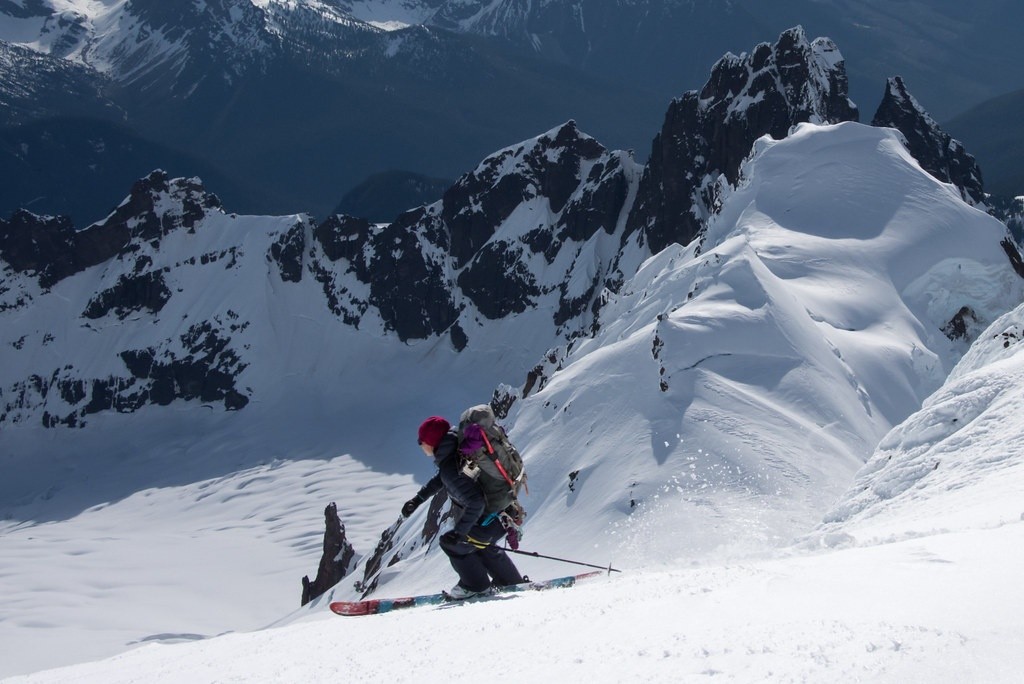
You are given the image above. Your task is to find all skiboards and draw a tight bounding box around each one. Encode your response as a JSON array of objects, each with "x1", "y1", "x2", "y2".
[{"x1": 330, "y1": 571, "x2": 605, "y2": 616}]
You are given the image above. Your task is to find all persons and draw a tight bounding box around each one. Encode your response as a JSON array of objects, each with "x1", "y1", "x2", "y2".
[{"x1": 401, "y1": 416, "x2": 527, "y2": 600}]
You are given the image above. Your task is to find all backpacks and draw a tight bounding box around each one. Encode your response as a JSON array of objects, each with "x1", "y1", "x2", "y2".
[{"x1": 455, "y1": 404, "x2": 525, "y2": 515}]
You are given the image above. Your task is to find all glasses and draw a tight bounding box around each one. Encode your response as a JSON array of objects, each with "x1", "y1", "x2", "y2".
[{"x1": 418, "y1": 439, "x2": 422, "y2": 445}]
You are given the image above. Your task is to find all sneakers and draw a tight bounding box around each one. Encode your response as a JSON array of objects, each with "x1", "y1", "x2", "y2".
[
  {"x1": 490, "y1": 579, "x2": 515, "y2": 590},
  {"x1": 451, "y1": 584, "x2": 490, "y2": 600}
]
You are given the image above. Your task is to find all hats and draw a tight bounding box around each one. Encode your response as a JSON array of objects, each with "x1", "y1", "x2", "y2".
[{"x1": 419, "y1": 417, "x2": 450, "y2": 449}]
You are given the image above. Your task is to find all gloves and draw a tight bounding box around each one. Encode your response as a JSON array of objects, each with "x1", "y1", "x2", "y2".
[
  {"x1": 440, "y1": 530, "x2": 462, "y2": 545},
  {"x1": 401, "y1": 495, "x2": 423, "y2": 518}
]
